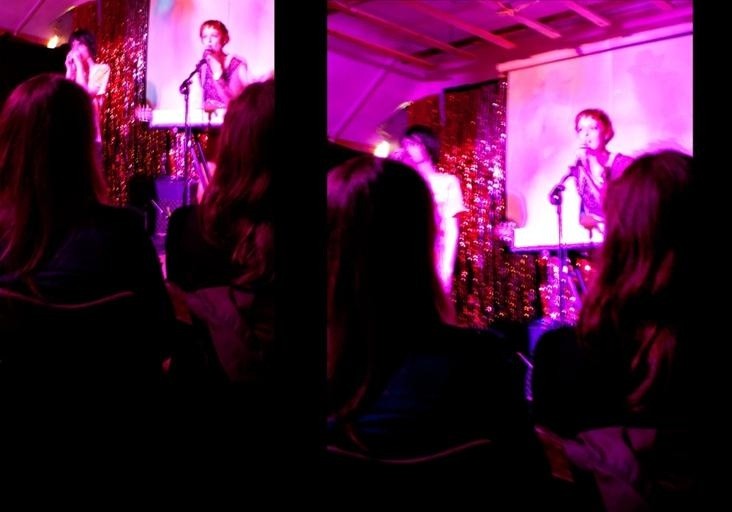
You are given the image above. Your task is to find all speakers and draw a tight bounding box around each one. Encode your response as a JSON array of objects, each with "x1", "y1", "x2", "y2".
[{"x1": 127, "y1": 175, "x2": 197, "y2": 252}]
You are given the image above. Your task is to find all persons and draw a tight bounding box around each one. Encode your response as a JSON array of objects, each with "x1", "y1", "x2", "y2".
[
  {"x1": 248, "y1": 160, "x2": 551, "y2": 512},
  {"x1": 188, "y1": 19, "x2": 257, "y2": 125},
  {"x1": 573, "y1": 106, "x2": 638, "y2": 243},
  {"x1": 63, "y1": 28, "x2": 113, "y2": 194},
  {"x1": 0, "y1": 72, "x2": 175, "y2": 501},
  {"x1": 167, "y1": 81, "x2": 314, "y2": 306},
  {"x1": 402, "y1": 126, "x2": 466, "y2": 320},
  {"x1": 552, "y1": 151, "x2": 703, "y2": 512},
  {"x1": 0, "y1": 72, "x2": 175, "y2": 512}
]
